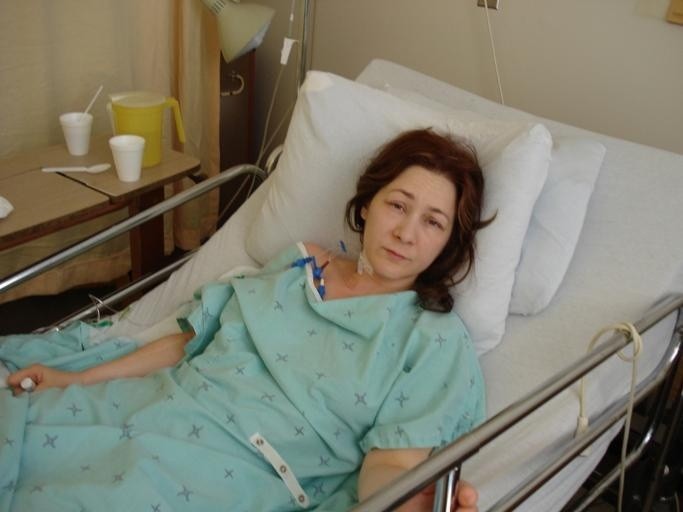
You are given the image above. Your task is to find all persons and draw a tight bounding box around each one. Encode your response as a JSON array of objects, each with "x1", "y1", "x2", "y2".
[{"x1": 0, "y1": 126, "x2": 499, "y2": 512}]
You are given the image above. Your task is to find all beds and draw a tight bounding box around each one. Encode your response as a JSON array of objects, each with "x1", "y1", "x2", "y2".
[{"x1": 25, "y1": 58, "x2": 683, "y2": 511}]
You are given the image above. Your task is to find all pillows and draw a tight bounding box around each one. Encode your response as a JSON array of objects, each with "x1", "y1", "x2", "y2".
[
  {"x1": 381, "y1": 83, "x2": 605, "y2": 317},
  {"x1": 244, "y1": 70, "x2": 553, "y2": 357}
]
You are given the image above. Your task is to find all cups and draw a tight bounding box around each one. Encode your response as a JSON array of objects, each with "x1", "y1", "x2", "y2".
[
  {"x1": 108, "y1": 135, "x2": 147, "y2": 185},
  {"x1": 59, "y1": 112, "x2": 97, "y2": 155}
]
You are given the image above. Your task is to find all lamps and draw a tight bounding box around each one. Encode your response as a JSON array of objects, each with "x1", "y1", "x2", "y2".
[{"x1": 198, "y1": 1, "x2": 275, "y2": 62}]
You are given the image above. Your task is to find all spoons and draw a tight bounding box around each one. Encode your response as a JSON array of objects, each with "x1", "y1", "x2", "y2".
[{"x1": 39, "y1": 159, "x2": 113, "y2": 174}]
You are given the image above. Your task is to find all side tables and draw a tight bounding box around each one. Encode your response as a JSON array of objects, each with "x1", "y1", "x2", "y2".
[{"x1": 0, "y1": 130, "x2": 201, "y2": 285}]
